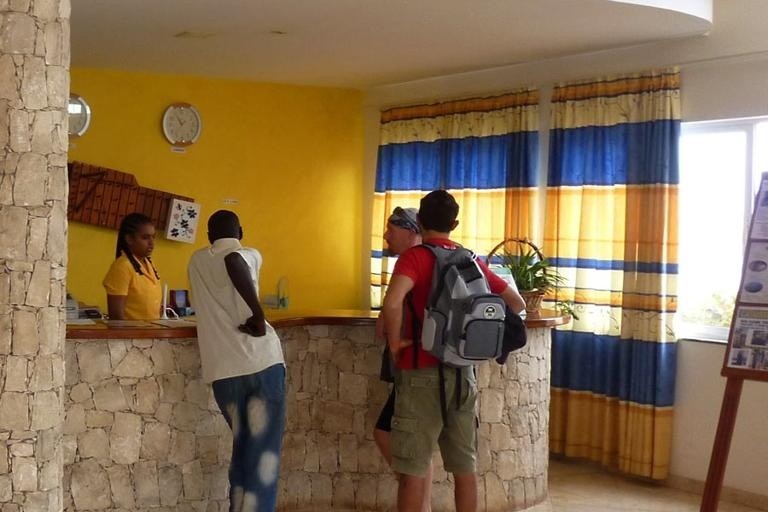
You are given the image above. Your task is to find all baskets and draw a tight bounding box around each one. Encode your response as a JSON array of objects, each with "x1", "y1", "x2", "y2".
[{"x1": 519, "y1": 291, "x2": 545, "y2": 313}]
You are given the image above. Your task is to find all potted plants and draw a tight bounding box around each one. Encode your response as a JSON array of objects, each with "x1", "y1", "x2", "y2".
[{"x1": 486, "y1": 239, "x2": 579, "y2": 322}]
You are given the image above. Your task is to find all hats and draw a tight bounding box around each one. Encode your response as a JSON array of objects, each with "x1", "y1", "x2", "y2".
[
  {"x1": 388, "y1": 207, "x2": 420, "y2": 233},
  {"x1": 495, "y1": 305, "x2": 526, "y2": 365}
]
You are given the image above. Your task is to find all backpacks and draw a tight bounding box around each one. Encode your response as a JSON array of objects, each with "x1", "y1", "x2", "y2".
[{"x1": 415, "y1": 244, "x2": 506, "y2": 368}]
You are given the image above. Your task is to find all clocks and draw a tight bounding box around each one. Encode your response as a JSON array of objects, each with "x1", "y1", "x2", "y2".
[
  {"x1": 69, "y1": 94, "x2": 91, "y2": 136},
  {"x1": 161, "y1": 102, "x2": 202, "y2": 147}
]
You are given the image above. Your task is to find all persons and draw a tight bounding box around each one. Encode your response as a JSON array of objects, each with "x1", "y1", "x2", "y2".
[
  {"x1": 187, "y1": 209, "x2": 287, "y2": 512},
  {"x1": 103, "y1": 213, "x2": 164, "y2": 319}
]
